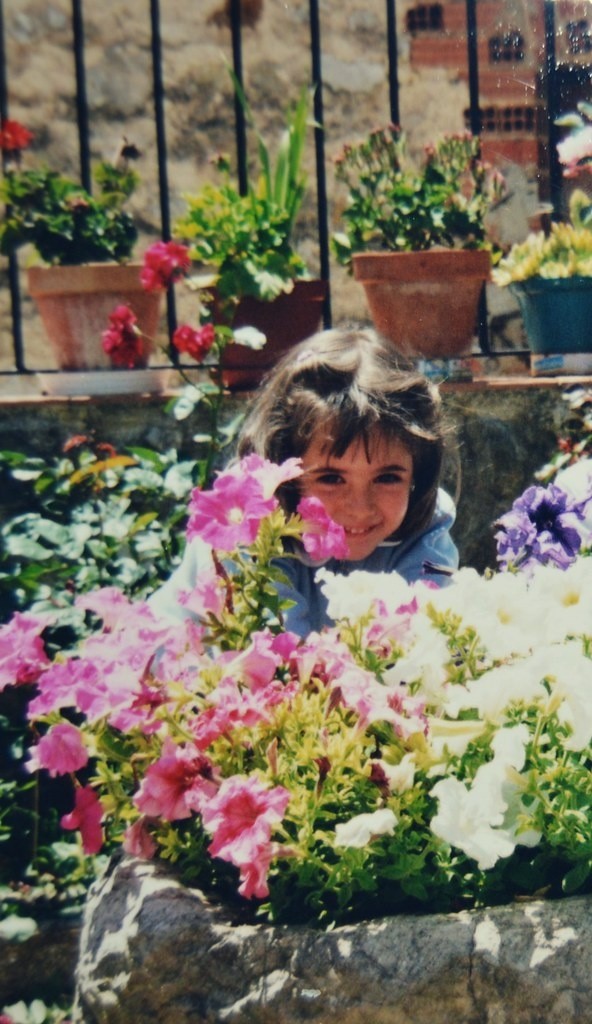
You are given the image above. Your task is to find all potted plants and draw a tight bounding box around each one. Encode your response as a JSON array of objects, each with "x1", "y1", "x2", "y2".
[
  {"x1": 170, "y1": 46, "x2": 329, "y2": 391},
  {"x1": 491, "y1": 188, "x2": 592, "y2": 378}
]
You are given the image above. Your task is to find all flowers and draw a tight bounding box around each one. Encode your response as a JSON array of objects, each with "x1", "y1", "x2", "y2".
[
  {"x1": 0, "y1": 452, "x2": 592, "y2": 899},
  {"x1": 0, "y1": 120, "x2": 144, "y2": 266},
  {"x1": 329, "y1": 119, "x2": 515, "y2": 277}
]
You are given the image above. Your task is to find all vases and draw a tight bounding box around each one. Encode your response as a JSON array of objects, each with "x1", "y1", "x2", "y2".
[
  {"x1": 352, "y1": 250, "x2": 493, "y2": 360},
  {"x1": 28, "y1": 263, "x2": 164, "y2": 372}
]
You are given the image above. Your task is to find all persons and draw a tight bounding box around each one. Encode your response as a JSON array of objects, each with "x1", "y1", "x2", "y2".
[{"x1": 133, "y1": 323, "x2": 461, "y2": 645}]
